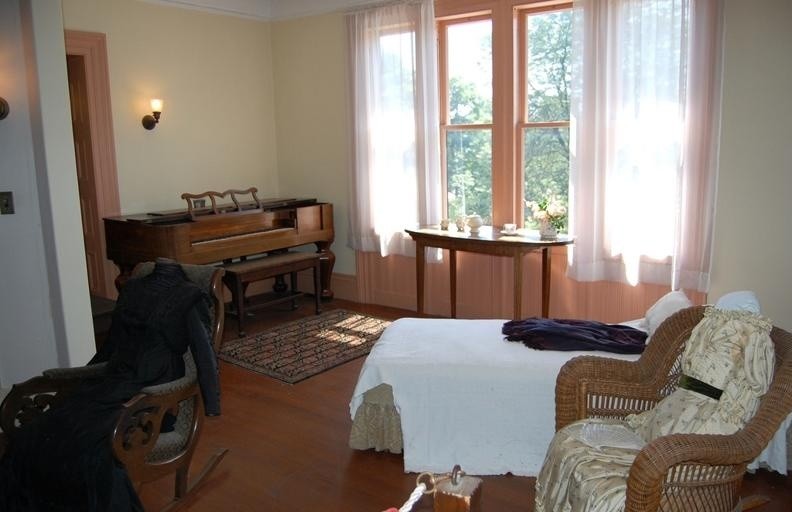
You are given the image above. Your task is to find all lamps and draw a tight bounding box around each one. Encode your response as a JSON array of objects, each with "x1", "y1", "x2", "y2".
[{"x1": 142, "y1": 99, "x2": 164, "y2": 130}]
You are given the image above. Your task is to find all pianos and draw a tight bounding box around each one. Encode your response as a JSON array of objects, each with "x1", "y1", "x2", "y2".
[{"x1": 101, "y1": 188, "x2": 335, "y2": 300}]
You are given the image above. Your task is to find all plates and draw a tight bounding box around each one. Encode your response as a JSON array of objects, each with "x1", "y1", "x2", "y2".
[{"x1": 500, "y1": 231, "x2": 521, "y2": 235}]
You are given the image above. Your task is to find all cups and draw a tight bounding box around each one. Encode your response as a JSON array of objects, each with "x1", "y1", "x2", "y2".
[
  {"x1": 504, "y1": 224, "x2": 516, "y2": 232},
  {"x1": 456, "y1": 218, "x2": 466, "y2": 232},
  {"x1": 441, "y1": 219, "x2": 449, "y2": 230}
]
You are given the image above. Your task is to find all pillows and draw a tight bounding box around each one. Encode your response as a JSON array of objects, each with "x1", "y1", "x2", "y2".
[
  {"x1": 641, "y1": 288, "x2": 692, "y2": 335},
  {"x1": 626, "y1": 305, "x2": 771, "y2": 444}
]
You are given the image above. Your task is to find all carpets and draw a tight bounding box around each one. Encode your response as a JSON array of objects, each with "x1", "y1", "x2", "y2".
[{"x1": 218, "y1": 309, "x2": 393, "y2": 384}]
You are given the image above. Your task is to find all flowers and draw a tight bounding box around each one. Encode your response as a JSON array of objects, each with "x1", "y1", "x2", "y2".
[{"x1": 523, "y1": 193, "x2": 566, "y2": 231}]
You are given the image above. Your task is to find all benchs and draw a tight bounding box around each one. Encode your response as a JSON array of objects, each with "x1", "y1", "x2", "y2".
[{"x1": 218, "y1": 252, "x2": 324, "y2": 337}]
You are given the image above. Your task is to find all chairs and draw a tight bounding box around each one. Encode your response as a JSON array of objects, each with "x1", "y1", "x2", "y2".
[
  {"x1": 1, "y1": 260, "x2": 234, "y2": 510},
  {"x1": 535, "y1": 306, "x2": 790, "y2": 511}
]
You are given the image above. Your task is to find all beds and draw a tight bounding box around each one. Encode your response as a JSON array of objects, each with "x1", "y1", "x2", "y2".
[{"x1": 349, "y1": 318, "x2": 652, "y2": 472}]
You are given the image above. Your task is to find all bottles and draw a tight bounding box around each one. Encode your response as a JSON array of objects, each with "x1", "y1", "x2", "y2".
[{"x1": 467, "y1": 213, "x2": 482, "y2": 232}]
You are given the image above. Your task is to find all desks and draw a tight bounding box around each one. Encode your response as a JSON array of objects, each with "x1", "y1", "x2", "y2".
[{"x1": 406, "y1": 224, "x2": 573, "y2": 320}]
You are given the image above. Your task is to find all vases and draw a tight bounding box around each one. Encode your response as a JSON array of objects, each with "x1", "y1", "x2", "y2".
[{"x1": 539, "y1": 221, "x2": 558, "y2": 238}]
